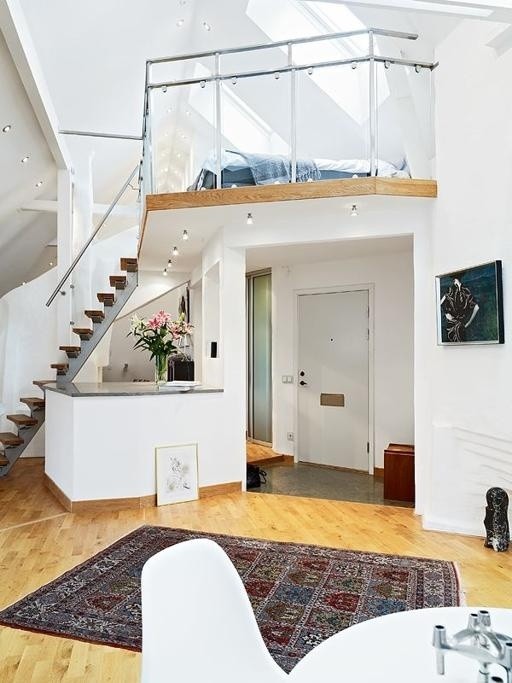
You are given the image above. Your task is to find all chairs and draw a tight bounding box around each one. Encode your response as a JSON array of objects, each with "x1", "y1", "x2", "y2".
[{"x1": 138, "y1": 537, "x2": 286, "y2": 680}]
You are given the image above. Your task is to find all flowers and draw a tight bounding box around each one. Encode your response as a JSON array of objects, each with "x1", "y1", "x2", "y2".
[{"x1": 124, "y1": 308, "x2": 196, "y2": 382}]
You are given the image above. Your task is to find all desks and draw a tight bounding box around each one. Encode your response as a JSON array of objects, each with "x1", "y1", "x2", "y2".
[{"x1": 283, "y1": 604, "x2": 512, "y2": 682}]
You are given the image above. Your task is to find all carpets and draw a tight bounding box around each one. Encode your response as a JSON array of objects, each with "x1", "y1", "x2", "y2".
[{"x1": 0, "y1": 520, "x2": 463, "y2": 676}]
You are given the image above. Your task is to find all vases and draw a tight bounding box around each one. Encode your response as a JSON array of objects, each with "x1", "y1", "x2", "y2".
[{"x1": 152, "y1": 351, "x2": 170, "y2": 390}]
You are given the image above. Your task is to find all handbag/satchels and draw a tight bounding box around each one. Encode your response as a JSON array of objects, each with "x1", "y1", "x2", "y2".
[{"x1": 247, "y1": 463, "x2": 267, "y2": 488}]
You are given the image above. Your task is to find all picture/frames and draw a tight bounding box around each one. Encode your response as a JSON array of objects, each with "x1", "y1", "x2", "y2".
[
  {"x1": 154, "y1": 442, "x2": 200, "y2": 507},
  {"x1": 434, "y1": 261, "x2": 505, "y2": 347}
]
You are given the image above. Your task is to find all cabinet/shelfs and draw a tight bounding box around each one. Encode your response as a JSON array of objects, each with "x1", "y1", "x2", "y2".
[{"x1": 383, "y1": 443, "x2": 414, "y2": 502}]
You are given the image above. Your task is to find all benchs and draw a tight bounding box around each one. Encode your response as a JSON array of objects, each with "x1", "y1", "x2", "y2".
[{"x1": 246, "y1": 441, "x2": 285, "y2": 472}]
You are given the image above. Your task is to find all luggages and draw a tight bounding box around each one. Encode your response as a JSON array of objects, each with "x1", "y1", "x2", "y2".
[{"x1": 168, "y1": 355, "x2": 194, "y2": 381}]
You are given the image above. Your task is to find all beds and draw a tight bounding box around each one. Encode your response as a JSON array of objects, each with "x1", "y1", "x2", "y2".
[{"x1": 185, "y1": 146, "x2": 411, "y2": 192}]
